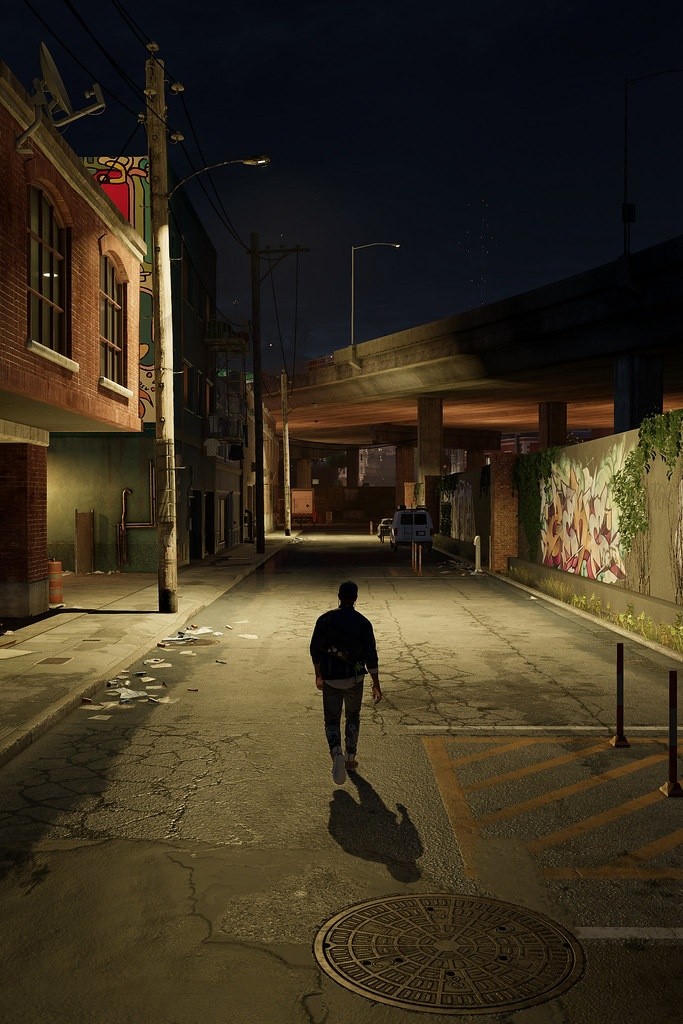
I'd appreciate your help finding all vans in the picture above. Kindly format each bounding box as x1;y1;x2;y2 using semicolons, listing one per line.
389;504;435;551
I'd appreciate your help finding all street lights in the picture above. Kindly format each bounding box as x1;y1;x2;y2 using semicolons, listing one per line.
346;238;406;357
164;155;278;195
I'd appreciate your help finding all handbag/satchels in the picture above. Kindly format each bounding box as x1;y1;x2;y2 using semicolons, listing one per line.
321;635;354;663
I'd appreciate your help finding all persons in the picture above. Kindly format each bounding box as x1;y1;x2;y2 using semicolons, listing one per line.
309;580;383;785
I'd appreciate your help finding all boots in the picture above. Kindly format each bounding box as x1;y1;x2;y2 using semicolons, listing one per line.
345;750;358;770
333;746;346;785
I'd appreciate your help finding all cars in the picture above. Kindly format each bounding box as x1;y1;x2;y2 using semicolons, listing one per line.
377;518;395;537
